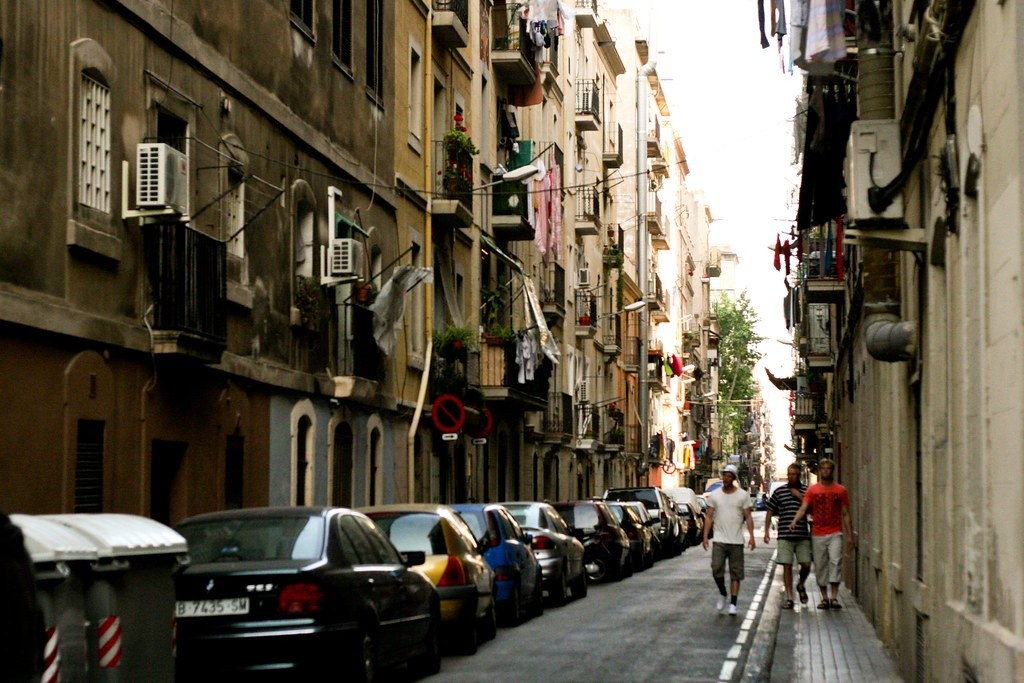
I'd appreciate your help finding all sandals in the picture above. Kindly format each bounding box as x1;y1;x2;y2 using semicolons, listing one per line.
830;598;841;609
817;599;829;609
797;584;808;603
783;599;794;609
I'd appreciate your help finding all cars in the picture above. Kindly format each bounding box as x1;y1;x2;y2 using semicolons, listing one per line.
169;504;445;682
497;499;589;607
446;501;543;629
549;485;770;584
353;505;498;657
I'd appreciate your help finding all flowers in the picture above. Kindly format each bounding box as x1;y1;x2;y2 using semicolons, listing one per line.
437;162;475;186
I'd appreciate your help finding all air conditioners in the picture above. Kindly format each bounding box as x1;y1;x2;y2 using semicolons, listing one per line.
806;301;830;356
843;118;903;221
794;393;814;417
578;380;590;402
332;237;364;277
135;143;190;213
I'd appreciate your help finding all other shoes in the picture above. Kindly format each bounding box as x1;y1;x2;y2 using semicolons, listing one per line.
728;604;737;615
717;594;728;610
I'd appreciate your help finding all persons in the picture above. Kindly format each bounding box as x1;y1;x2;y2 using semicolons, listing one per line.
789;458;854;610
764;464;810;609
703;464;756;615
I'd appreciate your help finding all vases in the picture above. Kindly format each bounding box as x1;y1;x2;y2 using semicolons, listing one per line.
445;178;467;192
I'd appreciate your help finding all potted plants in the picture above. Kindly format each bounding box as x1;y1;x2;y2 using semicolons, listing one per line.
484;325;513;346
444;130;479;161
433;322;474;352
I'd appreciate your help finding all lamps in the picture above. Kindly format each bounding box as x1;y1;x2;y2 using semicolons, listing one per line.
471;164;539;191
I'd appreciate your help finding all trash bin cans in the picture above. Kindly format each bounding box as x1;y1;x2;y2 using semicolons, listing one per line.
10;510;194;683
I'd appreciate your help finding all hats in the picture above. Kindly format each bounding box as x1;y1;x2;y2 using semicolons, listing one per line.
720;464;738;480
